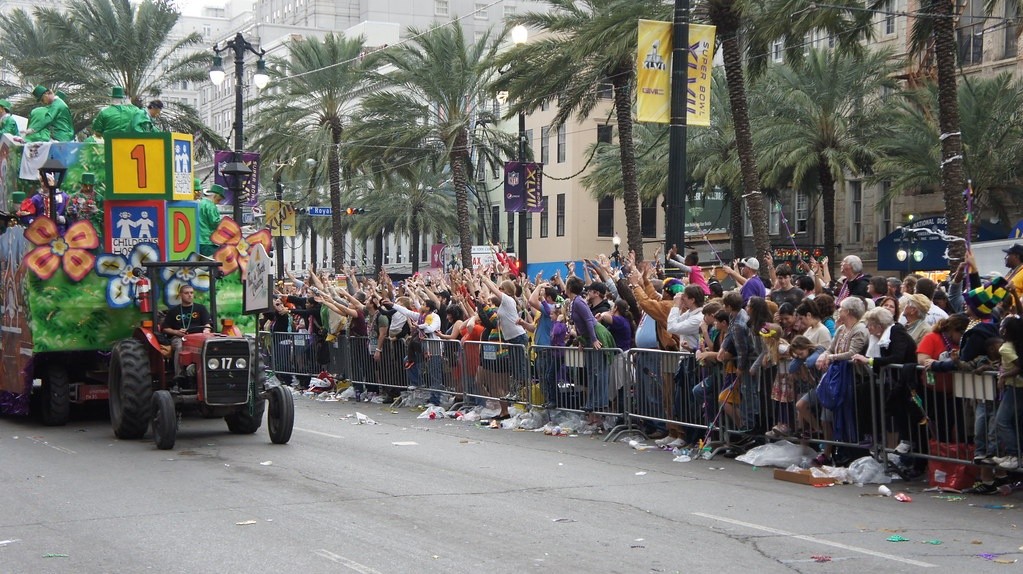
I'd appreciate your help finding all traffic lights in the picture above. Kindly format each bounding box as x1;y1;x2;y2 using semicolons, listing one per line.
346;207;364;215
294;207;306;215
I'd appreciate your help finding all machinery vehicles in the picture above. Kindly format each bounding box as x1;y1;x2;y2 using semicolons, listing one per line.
107;260;296;450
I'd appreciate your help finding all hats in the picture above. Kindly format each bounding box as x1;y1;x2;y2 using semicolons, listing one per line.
108;87;124;98
0;99;12;113
738;257;760;271
434;290;450;300
206;184;226;199
78;174;98;185
962;277;1009;318
663;277;685;296
33;84;49;102
309;285;319;296
904;292;932;315
1003;243;1023;255
584;282;606;294
194;179;203;191
12;192;26;202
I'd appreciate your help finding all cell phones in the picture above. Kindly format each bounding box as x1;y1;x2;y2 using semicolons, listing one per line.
434;330;438;333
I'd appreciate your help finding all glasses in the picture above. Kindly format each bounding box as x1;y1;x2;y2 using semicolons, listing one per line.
499;287;502;290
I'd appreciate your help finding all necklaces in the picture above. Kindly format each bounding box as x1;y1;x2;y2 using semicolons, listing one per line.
180;303;194;341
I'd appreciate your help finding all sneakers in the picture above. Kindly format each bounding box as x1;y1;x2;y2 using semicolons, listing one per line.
995;475;1009;492
667;437;688;448
962;479;997;495
655;435;676;446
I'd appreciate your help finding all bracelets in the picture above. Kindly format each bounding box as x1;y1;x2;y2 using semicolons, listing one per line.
869;358;874;366
375;348;382;352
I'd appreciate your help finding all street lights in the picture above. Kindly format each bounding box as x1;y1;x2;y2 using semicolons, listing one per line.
896;214;924;274
209;31;271;227
511;23;529;279
612;231;621;267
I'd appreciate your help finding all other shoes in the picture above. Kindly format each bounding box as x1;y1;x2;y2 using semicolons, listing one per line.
992;455;1018;469
894;440;911;454
812;453;832;467
492;414;510;420
170;381;180;394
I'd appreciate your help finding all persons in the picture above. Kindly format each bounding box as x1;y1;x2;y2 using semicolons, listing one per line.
84;87;163;142
0;84;74;142
8;191;26;216
1008;220;1023;239
194;177;203;201
16;173;68;237
162;285;216;394
68;172;104;239
259;241;1023;495
197;184;227;256
5;216;18;227
